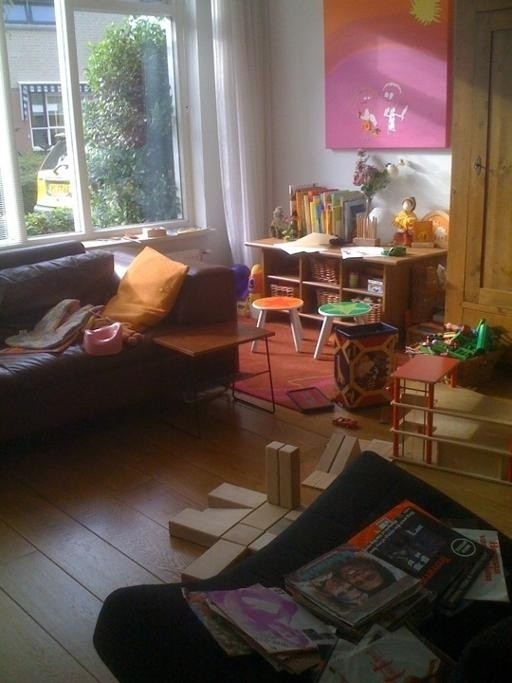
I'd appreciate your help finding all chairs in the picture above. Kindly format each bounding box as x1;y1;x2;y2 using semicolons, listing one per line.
92;451;512;683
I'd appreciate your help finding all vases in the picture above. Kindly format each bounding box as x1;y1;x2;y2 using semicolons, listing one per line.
352;211;381;247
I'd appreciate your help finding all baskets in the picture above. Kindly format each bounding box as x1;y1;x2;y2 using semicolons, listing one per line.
405;339;505;388
270;254;384;325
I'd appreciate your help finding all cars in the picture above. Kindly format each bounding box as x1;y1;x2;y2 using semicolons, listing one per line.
32;130;122;221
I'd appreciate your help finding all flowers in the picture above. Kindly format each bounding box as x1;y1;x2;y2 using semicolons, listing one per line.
352;147;388;211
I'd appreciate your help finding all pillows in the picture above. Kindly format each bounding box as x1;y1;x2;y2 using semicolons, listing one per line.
98;247;189;333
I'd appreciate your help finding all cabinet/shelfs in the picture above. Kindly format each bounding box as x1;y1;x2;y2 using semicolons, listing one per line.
245;236;447;341
391;354;512;488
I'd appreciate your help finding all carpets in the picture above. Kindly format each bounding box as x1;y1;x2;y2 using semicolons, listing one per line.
230;309;339;413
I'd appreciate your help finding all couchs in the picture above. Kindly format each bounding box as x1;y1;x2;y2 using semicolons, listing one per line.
1;242;250;446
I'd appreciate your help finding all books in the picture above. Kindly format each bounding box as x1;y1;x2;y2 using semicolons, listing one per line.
290;185;366;243
286;541;434;644
181;583;336;681
349;502;508;606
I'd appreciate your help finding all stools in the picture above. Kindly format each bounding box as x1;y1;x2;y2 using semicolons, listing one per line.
314;301;372;359
250;297;304;353
